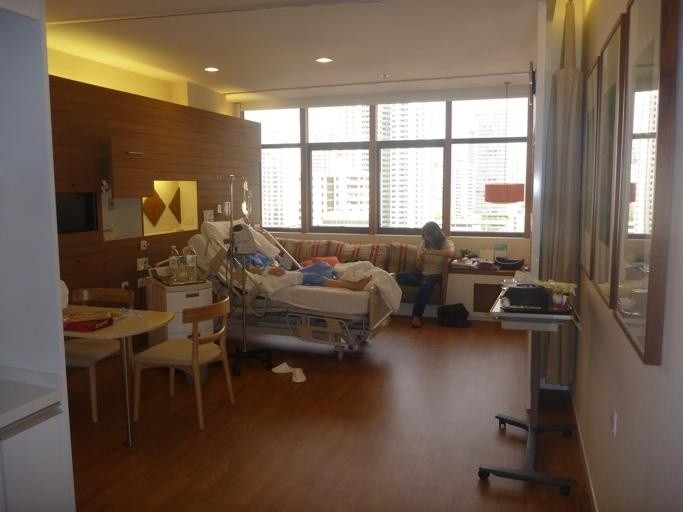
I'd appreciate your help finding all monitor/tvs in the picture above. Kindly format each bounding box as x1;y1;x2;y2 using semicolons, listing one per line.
55;192;98;234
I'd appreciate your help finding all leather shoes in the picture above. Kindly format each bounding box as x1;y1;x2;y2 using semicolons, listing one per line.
411;318;423;328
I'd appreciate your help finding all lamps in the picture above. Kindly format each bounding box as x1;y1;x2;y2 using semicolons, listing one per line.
482;180;524;268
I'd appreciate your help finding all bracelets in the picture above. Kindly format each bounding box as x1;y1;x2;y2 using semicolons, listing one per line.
428;249;432;255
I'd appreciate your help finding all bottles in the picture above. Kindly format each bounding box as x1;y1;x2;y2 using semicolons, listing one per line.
242;190;252;219
186;244;198;283
169;245;179;280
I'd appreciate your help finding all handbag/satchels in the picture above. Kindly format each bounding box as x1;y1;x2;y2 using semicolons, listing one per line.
437;303;472;326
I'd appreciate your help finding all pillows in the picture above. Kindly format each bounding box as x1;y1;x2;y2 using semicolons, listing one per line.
200;216;269;246
273;238;418;275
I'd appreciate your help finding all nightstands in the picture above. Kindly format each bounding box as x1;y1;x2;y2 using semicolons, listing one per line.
144;277;215;348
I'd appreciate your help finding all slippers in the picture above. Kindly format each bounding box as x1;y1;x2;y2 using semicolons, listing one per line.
292;367;306;384
271;362;293;374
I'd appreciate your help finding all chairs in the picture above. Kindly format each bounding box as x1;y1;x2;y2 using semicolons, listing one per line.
67;284;237;449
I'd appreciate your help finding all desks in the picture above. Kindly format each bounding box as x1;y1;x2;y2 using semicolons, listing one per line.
445;258;528;319
475;279;582;497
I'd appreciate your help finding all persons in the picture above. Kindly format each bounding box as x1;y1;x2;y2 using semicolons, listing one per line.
393;221;456;329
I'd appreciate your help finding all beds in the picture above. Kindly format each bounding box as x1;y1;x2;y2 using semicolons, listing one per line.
169;219;398;379
